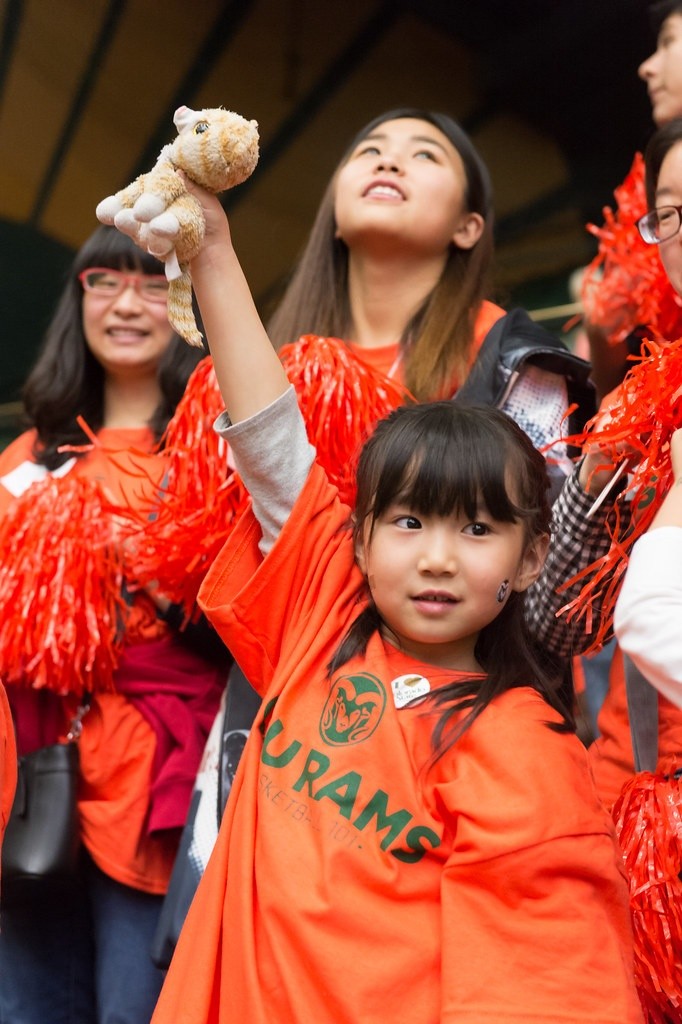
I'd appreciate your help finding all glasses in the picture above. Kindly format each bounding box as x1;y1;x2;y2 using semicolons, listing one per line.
635;205;682;245
80;268;169;304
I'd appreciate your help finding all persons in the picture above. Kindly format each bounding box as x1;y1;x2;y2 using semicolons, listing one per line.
0;9;680;1022
153;181;649;1024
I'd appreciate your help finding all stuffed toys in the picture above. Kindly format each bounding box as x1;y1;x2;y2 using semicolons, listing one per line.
95;104;260;348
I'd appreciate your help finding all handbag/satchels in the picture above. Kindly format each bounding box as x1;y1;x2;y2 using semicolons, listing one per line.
2;745;83;879
